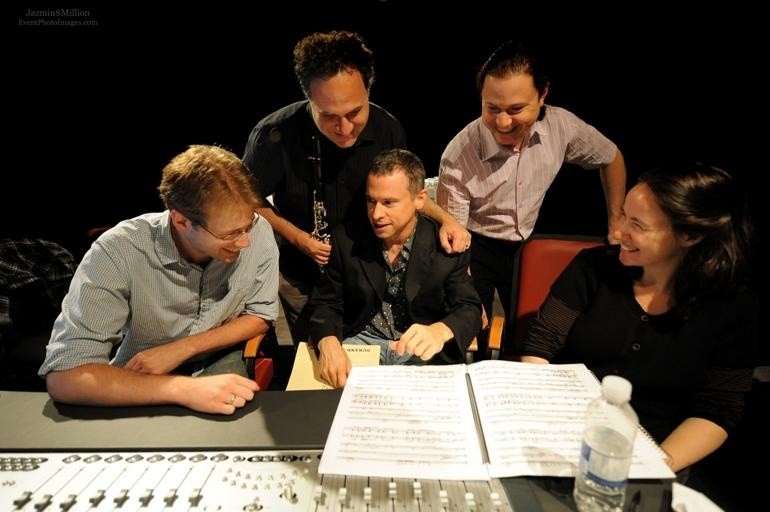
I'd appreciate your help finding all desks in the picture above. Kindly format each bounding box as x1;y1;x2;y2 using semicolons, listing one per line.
0;388;677;512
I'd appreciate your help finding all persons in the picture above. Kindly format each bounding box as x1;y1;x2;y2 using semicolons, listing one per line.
302;148;483;389
435;46;628;362
241;31;409;343
38;144;284;416
520;162;759;493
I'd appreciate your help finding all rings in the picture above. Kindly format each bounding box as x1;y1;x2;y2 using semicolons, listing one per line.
226;393;236;403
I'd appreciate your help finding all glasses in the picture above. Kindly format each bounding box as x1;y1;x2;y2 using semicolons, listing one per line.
194;211;260;240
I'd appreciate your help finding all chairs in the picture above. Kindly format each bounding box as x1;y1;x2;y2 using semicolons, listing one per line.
466;338;479;365
502;234;608;360
242;326;284;391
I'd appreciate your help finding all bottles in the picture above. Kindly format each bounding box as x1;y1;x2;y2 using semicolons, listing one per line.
571;371;641;512
